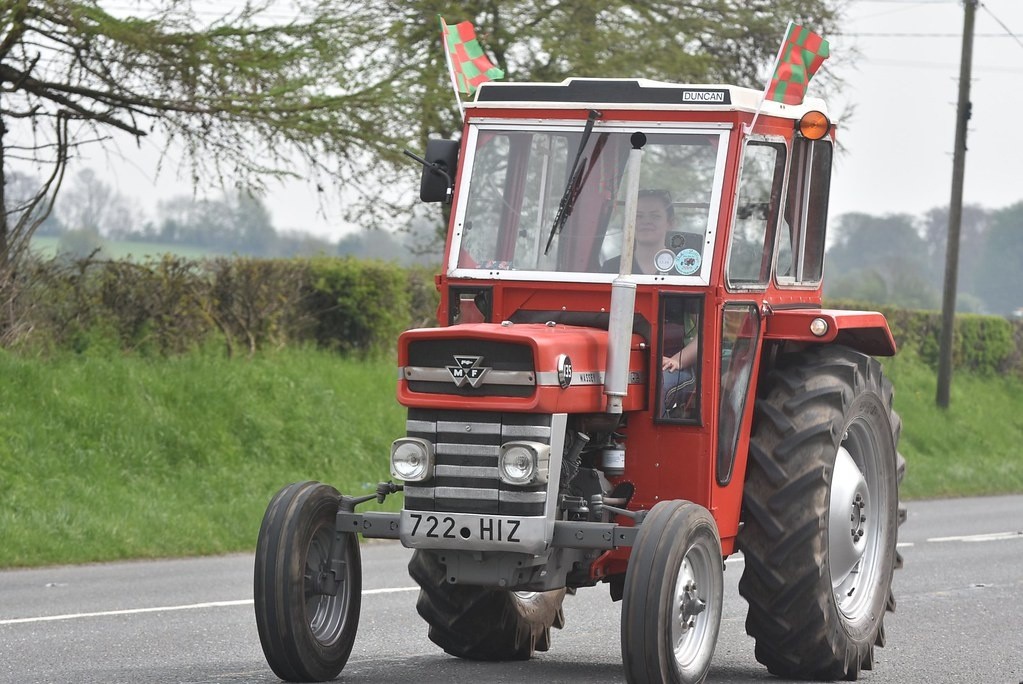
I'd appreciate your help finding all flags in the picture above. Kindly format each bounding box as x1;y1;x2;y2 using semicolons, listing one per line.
438;17;507;96
764;23;830;106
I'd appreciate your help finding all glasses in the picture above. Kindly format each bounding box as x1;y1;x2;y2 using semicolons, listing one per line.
638;189;673;204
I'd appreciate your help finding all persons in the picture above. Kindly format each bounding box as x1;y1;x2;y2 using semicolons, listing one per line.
600;189;698;416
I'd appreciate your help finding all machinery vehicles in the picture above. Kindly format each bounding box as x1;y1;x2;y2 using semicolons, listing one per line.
254;77;909;684
